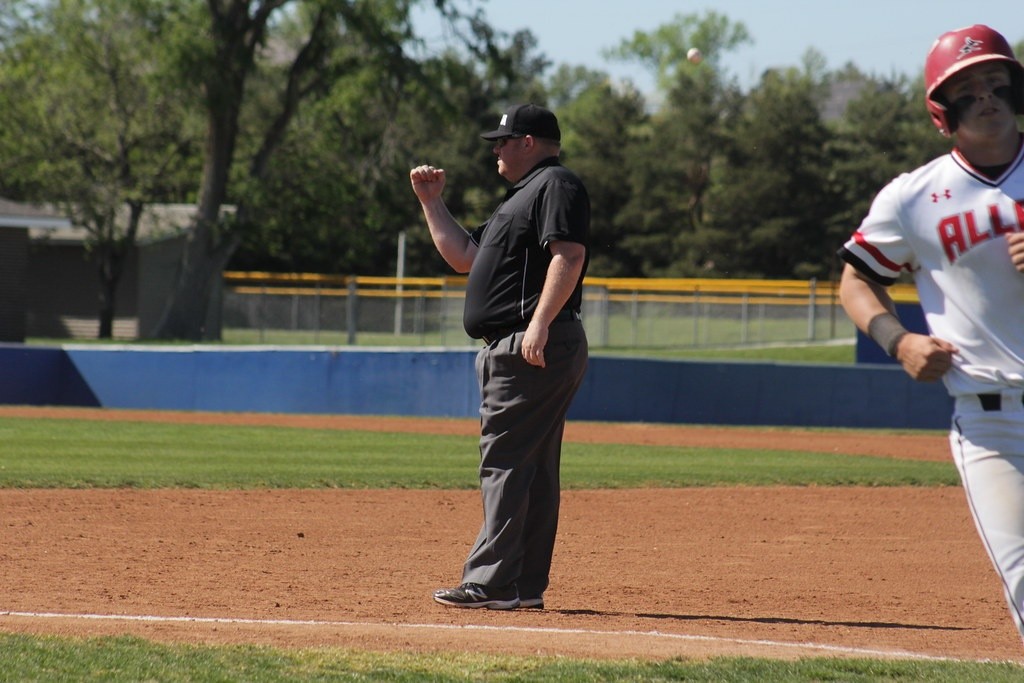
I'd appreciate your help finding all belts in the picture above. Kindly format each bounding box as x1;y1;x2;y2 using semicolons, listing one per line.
976;394;1003;412
481;312;581;345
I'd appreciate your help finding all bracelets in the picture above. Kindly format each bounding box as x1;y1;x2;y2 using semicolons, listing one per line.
869;313;907;359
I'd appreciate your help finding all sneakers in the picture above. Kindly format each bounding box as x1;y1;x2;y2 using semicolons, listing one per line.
519;593;544;607
433;582;520;610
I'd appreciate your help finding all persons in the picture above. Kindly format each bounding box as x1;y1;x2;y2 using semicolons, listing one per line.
408;103;587;613
837;24;1024;648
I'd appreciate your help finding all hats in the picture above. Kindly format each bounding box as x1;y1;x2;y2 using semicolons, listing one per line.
480;102;561;141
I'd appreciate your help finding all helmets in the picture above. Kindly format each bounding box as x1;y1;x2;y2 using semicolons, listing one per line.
926;25;1024;139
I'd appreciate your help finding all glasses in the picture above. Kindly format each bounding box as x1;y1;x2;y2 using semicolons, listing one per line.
497;136;524;147
